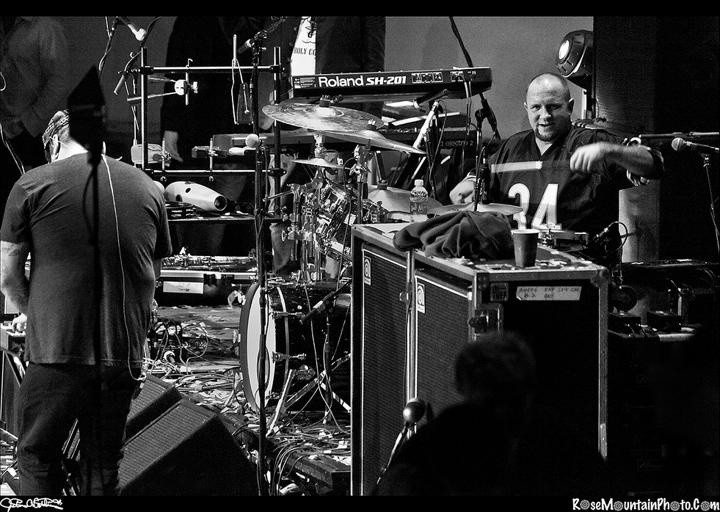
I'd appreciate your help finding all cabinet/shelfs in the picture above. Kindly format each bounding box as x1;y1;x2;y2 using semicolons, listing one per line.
347;220;613;497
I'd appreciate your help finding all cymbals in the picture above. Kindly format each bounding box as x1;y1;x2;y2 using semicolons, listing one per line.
292;159;351;171
262;102;384;133
349;185;447;222
427;201;522;217
306;129;427;154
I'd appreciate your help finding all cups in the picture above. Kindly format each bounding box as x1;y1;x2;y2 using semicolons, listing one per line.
510;228;541;268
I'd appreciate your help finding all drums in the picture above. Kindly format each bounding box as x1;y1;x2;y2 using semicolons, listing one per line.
238;278;351;417
303;181;392;269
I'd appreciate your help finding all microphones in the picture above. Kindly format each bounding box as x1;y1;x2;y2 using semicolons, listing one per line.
238;34;258;55
481;149;491;205
671;138;719;154
175;79;188;96
117;15;146;41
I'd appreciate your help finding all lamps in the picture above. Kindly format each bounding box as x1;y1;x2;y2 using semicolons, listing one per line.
555;29;594;119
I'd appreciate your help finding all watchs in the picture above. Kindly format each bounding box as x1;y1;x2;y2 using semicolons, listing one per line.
457;191;471;203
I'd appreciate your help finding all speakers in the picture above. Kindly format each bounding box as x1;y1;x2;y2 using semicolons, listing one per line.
114;399;254;499
63;376;182;473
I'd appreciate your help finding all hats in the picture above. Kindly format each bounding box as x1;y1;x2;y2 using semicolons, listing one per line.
41;108;69;162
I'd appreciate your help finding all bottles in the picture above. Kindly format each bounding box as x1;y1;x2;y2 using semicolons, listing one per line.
409;178;428;223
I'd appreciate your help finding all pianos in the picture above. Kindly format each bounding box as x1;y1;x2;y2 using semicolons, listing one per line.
270;68;493;105
1;319;26;353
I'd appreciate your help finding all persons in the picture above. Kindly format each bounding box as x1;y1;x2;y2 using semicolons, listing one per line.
159;16;266;308
0;109;173;497
369;330;608;494
449;72;665;365
0;17;73;197
267;16;386;281
